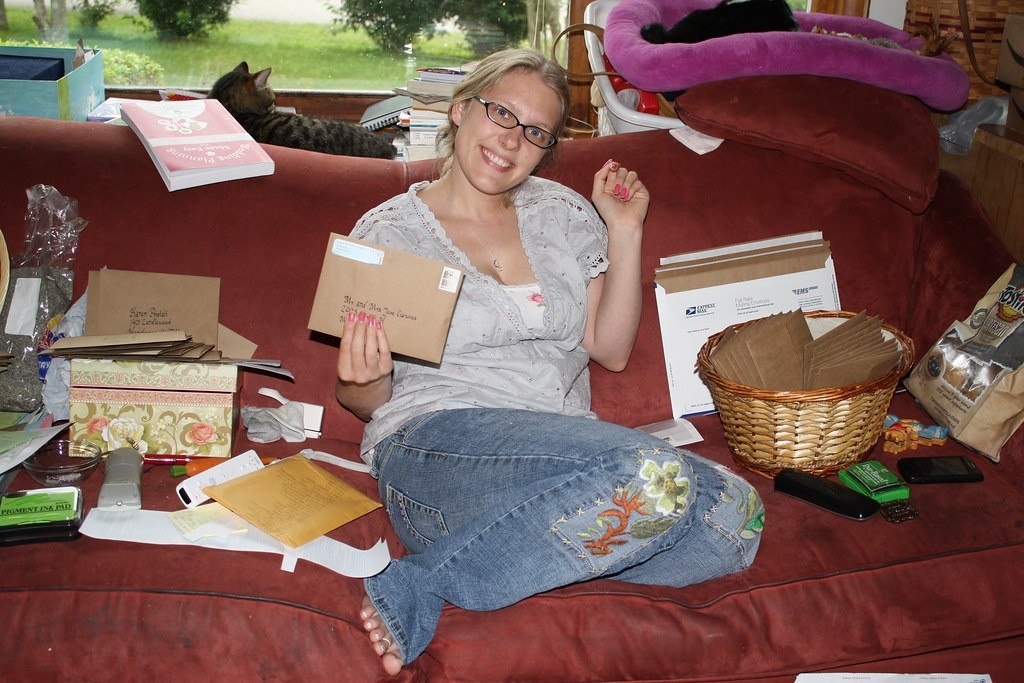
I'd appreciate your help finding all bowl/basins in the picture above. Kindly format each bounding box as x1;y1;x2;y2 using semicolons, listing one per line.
21;439;103;487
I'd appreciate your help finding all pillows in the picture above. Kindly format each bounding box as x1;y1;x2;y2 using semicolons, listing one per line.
675;75;942;214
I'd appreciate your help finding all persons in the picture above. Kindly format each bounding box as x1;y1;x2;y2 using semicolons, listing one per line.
335;48;765;676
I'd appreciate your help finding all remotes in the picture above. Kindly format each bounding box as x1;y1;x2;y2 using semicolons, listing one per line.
97;447;145;510
773;468;881;520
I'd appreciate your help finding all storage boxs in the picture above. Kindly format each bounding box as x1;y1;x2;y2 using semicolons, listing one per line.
583;0;685;133
69;357;243;459
0;45;105;121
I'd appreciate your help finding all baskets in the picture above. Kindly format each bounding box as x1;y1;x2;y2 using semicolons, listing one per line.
694;309;914;477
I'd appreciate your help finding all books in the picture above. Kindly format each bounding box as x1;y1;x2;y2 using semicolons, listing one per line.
359;67;475;162
121;99;275;192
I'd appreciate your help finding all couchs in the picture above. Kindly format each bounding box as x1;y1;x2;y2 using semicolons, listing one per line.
0;116;1024;682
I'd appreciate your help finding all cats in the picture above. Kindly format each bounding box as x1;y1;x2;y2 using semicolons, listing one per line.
205;61;397;160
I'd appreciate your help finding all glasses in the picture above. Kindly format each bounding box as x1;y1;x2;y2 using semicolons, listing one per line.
475;94;561;149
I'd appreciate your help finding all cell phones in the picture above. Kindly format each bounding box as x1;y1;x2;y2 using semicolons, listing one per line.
897;455;984;484
